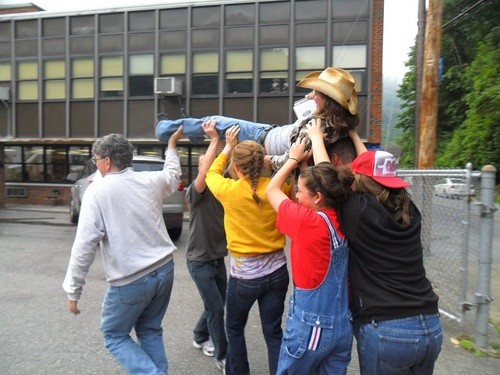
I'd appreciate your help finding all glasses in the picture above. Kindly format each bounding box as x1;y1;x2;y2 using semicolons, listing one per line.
91;155;104;164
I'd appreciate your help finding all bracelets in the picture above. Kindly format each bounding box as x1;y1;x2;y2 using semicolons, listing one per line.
289;158;300;164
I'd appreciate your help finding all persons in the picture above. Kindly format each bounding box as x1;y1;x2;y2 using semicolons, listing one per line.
156;67;360;172
63;124;186;375
184;118;443;375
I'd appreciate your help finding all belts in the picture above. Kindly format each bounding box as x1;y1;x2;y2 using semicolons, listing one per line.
259;123;278;144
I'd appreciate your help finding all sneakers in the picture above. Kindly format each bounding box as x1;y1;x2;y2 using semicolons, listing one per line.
214;358;227;375
192;338;215;357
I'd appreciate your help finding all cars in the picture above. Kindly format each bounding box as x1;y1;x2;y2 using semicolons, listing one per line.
435;178;476;202
66;155;185;239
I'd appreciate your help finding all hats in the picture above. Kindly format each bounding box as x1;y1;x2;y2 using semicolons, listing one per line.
295;66;359;116
351;149;412;190
